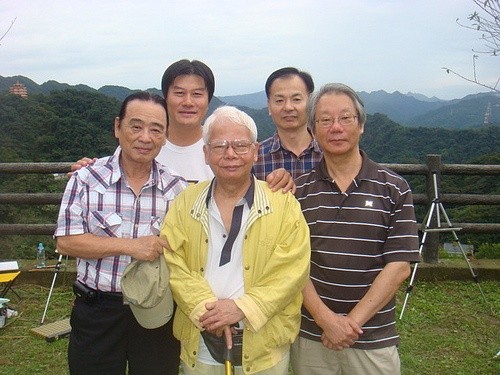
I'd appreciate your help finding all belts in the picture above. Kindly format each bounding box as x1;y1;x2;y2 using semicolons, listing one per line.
95;292;123;305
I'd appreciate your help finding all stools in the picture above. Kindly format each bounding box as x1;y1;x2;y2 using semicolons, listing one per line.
0;271;21;300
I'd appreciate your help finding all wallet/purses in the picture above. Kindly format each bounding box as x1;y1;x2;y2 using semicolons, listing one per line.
198;324;244;365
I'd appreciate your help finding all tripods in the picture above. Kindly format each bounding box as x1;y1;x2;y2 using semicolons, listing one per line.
397;170;488;319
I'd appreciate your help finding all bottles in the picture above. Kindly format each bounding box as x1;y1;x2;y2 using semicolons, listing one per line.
36;243;45;269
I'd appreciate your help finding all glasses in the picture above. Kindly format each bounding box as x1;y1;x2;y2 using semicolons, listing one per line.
209;138;253;155
314;111;359;129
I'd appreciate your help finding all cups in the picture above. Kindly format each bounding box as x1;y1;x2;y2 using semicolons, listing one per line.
0;299;10;328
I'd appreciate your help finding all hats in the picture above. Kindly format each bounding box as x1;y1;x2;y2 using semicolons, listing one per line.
118;252;174;329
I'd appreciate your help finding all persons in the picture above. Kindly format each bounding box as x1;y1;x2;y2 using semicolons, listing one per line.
251;67;324;182
53;92;296;375
290;83;422;375
70;59;216;188
159;105;311;375
